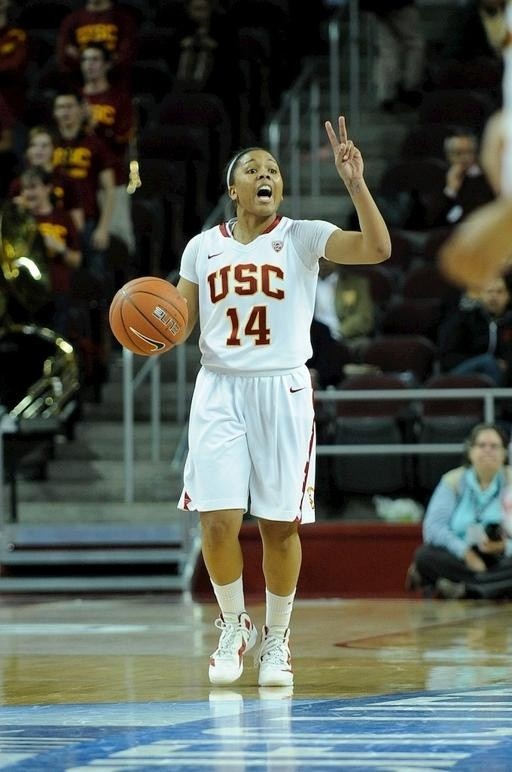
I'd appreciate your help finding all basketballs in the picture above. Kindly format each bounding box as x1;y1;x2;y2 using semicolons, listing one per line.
109;276;188;356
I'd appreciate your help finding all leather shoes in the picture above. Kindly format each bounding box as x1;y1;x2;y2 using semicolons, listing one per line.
404;563;424;592
435;578;487;601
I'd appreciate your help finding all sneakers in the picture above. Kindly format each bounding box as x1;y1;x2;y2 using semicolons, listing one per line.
258;624;294;687
208;611;258;685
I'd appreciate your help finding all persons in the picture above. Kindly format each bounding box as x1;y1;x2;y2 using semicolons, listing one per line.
407;420;510;598
1;0;509;515
176;115;392;690
